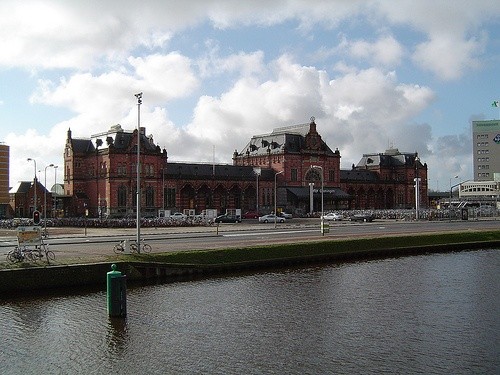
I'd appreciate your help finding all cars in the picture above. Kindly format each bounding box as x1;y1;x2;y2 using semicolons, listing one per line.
281;212;290;219
144;214;155;219
321;213;344;221
215;215;242;224
170;212;186;219
258;214;286;223
350;213;373;222
242;211;260;219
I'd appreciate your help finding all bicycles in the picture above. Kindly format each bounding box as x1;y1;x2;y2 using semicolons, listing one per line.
113;237;152;254
5;236;57;264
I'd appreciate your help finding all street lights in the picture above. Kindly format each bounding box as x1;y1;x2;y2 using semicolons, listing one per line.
313;165;324;233
274;170;285;229
449;176;460;222
27;158;38;211
43;164;54;237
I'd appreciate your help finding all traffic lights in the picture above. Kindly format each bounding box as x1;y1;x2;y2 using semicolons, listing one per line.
33;211;40;225
